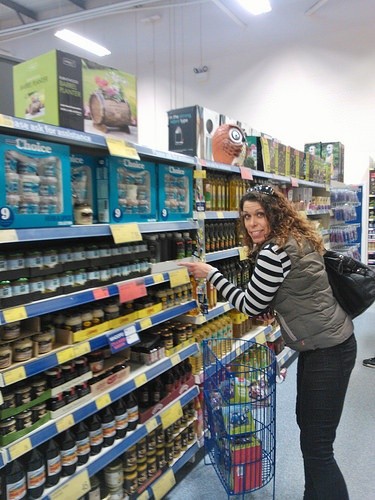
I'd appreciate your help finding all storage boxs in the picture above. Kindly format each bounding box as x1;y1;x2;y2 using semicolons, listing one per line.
221;377;248;406
214;432;262;494
218;404;255;435
0;49;344;228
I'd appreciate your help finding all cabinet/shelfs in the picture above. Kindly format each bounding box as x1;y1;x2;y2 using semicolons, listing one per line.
0;114;375;500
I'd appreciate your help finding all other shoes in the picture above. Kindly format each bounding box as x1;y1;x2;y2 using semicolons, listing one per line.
362;357;375;367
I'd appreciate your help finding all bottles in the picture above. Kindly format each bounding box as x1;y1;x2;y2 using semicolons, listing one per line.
140;171;286;439
0;357;192;500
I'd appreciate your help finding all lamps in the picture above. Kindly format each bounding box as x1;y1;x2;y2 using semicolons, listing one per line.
193;66;208;83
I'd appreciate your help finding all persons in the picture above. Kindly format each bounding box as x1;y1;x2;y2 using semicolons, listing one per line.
177;186;358;500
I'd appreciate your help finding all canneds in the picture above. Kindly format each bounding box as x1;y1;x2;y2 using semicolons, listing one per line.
0;284;193;436
79;398;195;500
4;151;187;225
0;241;154;308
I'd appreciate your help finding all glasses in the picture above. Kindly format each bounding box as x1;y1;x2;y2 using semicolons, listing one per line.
246;186;279;198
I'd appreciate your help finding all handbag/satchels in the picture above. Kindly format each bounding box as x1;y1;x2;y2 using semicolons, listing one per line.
323;250;375;320
